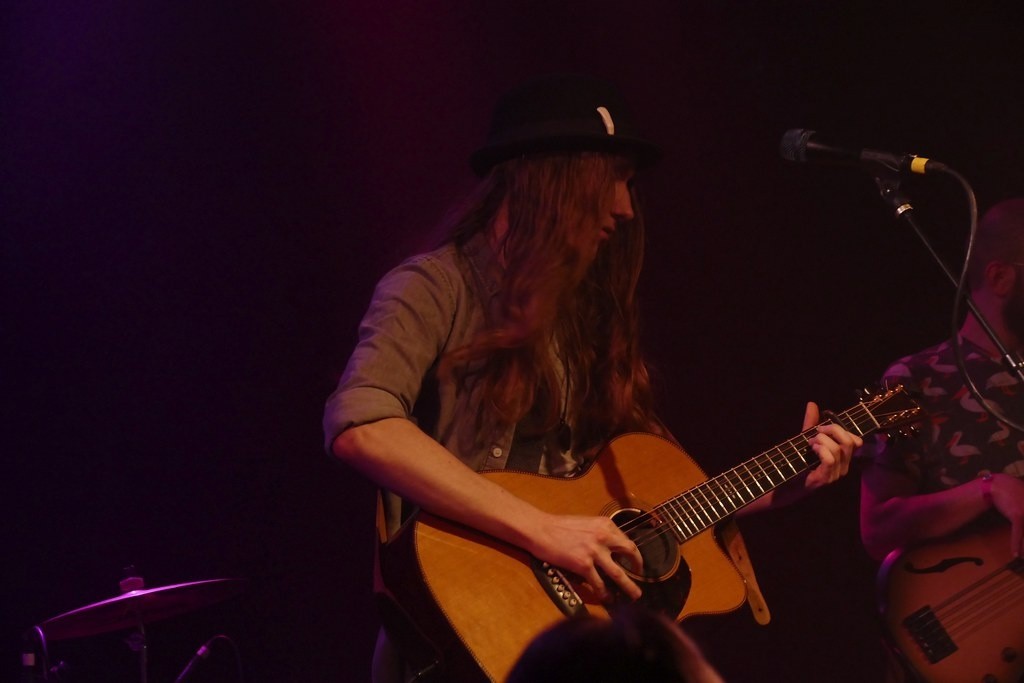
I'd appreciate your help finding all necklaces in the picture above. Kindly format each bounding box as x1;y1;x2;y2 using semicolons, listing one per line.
556;316;573;451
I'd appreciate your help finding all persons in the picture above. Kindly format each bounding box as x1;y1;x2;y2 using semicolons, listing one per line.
319;72;864;683
858;198;1024;683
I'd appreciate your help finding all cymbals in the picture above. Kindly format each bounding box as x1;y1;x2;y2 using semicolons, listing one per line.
23;574;250;642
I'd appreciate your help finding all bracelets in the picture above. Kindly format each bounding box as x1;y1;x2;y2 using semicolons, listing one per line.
983;471;992;491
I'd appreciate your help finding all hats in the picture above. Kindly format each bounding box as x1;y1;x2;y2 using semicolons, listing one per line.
471;65;662;170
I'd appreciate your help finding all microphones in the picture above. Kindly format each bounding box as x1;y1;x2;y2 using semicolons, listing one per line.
176;638;216;683
20;630;40;683
780;128;948;176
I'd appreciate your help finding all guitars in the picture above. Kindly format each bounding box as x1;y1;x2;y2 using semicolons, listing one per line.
375;377;928;683
868;473;1024;683
